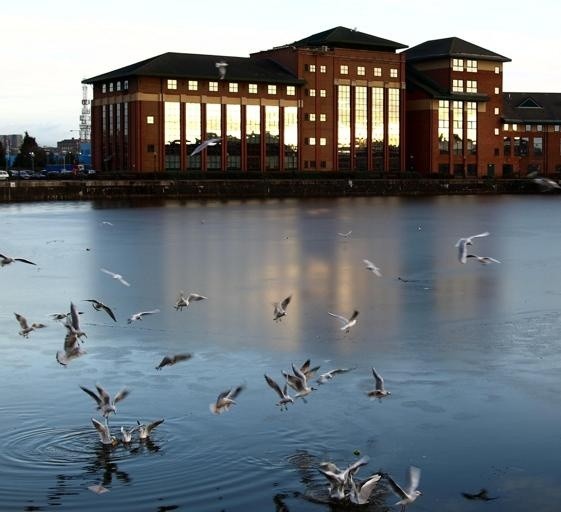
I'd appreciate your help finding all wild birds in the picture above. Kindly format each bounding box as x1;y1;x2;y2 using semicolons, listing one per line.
101;267;129;286
155;353;191;371
455;231;490;264
127;310;160;329
215;60;228;81
0;252;37;268
467;253;502;265
82;299;117;323
533;177;560;189
318;455;424;512
78;382;164;447
103;221;113;226
272;295;292;323
13;312;47;339
174;293;208;311
264;359;349;413
49;300;88;366
338;231;352;239
210;385;244;415
192;136;222;157
459;489;500;502
365;367;392;401
362;259;382;278
327;310;360;335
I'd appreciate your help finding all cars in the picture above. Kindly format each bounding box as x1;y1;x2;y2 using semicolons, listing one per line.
0;165;98;181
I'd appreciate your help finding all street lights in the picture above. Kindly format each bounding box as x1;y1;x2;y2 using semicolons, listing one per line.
70;128;82;165
29;151;34;176
62;150;66;175
